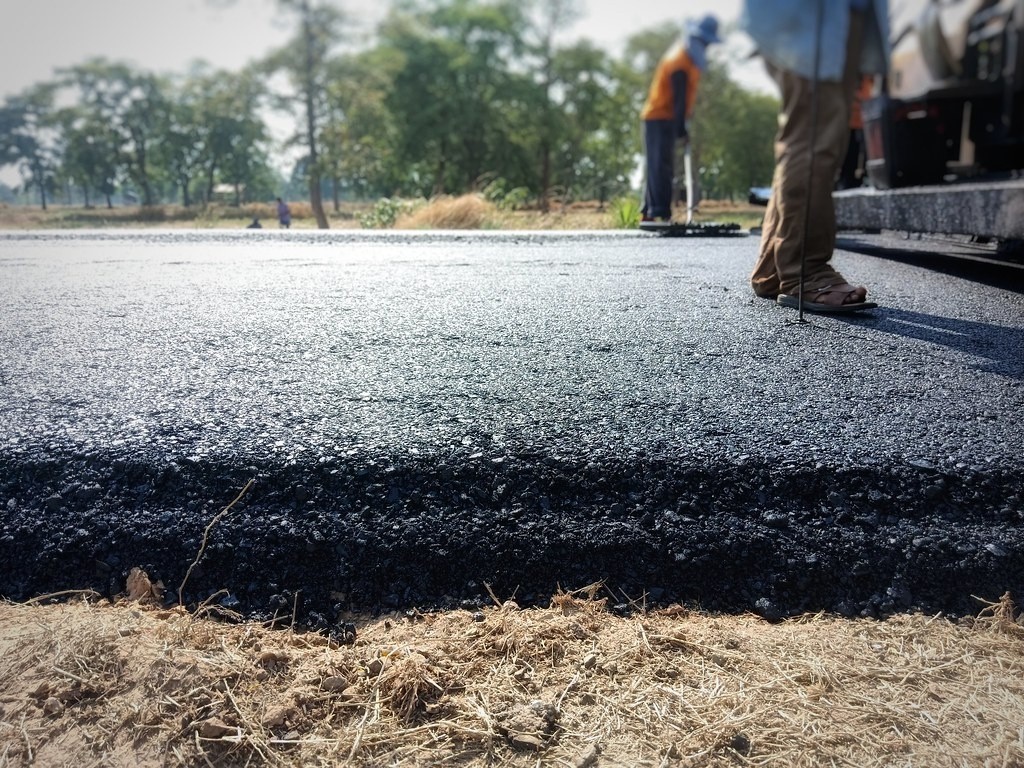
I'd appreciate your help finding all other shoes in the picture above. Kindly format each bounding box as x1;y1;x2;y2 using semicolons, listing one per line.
639;216;657;225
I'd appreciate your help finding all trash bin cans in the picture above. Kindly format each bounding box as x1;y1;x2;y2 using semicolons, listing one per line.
881;101;948;188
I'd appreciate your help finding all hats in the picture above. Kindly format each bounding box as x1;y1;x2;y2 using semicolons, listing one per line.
682;15;723;45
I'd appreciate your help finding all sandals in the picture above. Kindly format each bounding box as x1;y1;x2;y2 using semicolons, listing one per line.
776;281;878;314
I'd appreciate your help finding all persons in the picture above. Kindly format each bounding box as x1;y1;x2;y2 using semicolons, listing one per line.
638;16;724;224
276;197;291;228
743;0;892;313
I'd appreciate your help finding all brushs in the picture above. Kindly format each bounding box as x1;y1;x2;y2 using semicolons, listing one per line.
640;121;741;235
